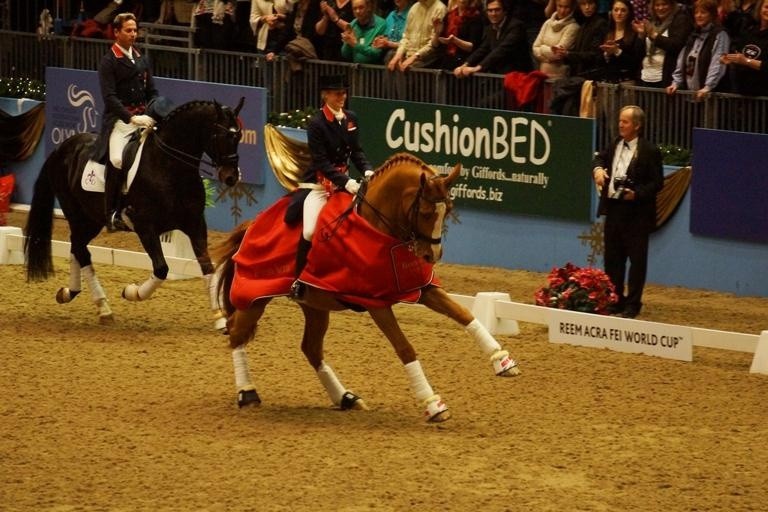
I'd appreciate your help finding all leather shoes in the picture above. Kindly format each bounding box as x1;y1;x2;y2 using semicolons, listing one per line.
623;304;643;318
606;299;627;314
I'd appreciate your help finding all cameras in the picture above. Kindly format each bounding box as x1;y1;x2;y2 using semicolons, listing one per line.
614;176;633;191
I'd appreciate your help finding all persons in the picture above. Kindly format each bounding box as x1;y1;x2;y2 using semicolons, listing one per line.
73;0;768;145
288;73;376;300
98;12;160;232
590;105;666;318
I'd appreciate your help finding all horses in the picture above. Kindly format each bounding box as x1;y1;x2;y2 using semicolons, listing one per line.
197;152;523;424
22;96;246;336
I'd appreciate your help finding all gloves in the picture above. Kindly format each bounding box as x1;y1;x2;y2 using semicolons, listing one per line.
344;178;361;195
130;114;158;127
364;169;375;182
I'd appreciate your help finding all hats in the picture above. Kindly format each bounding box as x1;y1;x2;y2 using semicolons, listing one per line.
318;74;352;90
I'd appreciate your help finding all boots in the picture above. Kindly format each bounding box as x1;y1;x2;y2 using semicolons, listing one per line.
103;159;125;233
290;232;312;299
